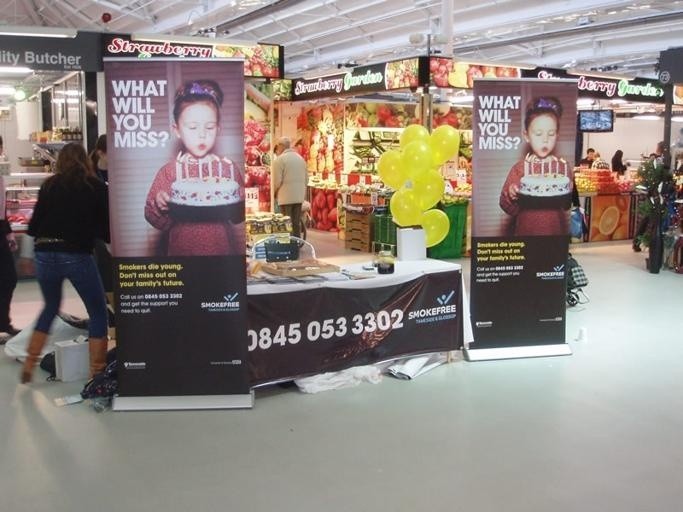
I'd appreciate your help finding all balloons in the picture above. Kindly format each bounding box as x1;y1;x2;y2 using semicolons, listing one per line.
378;124;460;249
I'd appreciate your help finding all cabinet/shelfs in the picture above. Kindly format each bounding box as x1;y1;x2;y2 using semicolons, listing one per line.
2;188;40;233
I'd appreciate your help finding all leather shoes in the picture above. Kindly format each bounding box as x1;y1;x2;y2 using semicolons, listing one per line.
0;324;21;335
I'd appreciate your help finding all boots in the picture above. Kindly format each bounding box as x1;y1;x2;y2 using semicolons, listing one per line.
21;330;48;385
87;336;107;382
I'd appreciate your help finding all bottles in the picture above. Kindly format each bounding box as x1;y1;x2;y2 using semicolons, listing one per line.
31;128;83;142
377;251;394;274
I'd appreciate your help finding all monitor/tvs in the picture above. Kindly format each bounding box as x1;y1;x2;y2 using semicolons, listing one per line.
577;109;614;133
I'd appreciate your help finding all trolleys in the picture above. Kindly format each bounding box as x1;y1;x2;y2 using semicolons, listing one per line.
566;253;590;306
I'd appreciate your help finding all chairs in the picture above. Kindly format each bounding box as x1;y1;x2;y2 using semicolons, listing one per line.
252;236;315;264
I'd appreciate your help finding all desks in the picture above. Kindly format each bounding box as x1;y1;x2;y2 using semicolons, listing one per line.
245;258;462;396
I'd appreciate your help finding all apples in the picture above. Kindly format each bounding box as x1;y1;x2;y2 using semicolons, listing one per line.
312;190;340;232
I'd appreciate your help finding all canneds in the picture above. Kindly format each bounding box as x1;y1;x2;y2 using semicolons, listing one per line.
246;213;293;234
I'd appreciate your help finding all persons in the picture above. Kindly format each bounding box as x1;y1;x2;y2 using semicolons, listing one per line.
21;143;109;382
144;80;246;257
87;135;115;339
499;97;575;239
578;142;665;252
272;137;309;244
0;135;23;345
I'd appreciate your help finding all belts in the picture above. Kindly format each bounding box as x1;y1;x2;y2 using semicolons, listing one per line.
33;237;64;245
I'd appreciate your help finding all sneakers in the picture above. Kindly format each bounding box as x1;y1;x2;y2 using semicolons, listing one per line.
632;244;641;251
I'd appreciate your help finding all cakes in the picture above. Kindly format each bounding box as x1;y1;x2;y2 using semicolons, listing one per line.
519;171;573;198
168;176;240;207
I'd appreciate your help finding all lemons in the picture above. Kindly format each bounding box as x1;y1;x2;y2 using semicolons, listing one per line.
575;178;598;193
